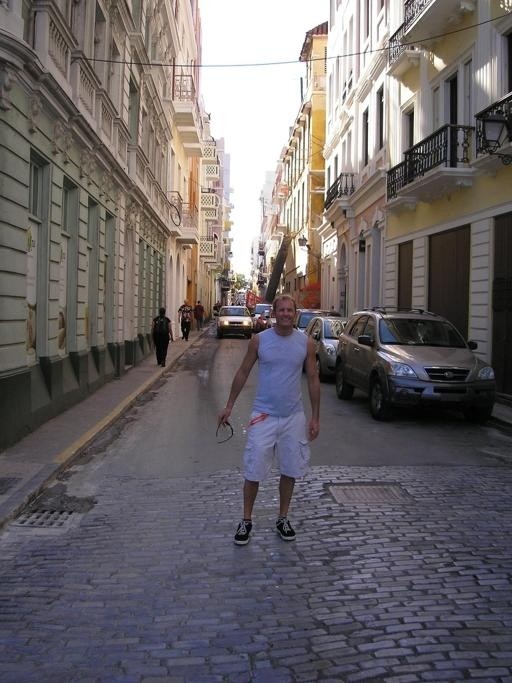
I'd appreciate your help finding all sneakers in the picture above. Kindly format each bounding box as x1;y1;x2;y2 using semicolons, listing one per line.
274;514;297;542
233;519;254;547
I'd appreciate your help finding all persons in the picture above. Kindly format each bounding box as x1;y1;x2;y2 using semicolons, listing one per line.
217;301;221;308
213;303;219;319
178;299;193;341
194;301;205;331
256;311;268;331
151;308;173;367
218;295;320;545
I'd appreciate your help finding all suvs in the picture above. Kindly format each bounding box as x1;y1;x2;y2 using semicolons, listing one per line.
333;305;502;427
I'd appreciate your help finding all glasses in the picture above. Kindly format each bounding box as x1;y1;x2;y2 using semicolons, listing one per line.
215;420;234;445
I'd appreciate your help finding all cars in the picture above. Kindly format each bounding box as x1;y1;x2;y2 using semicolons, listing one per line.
209;301;343;340
303;313;350;384
